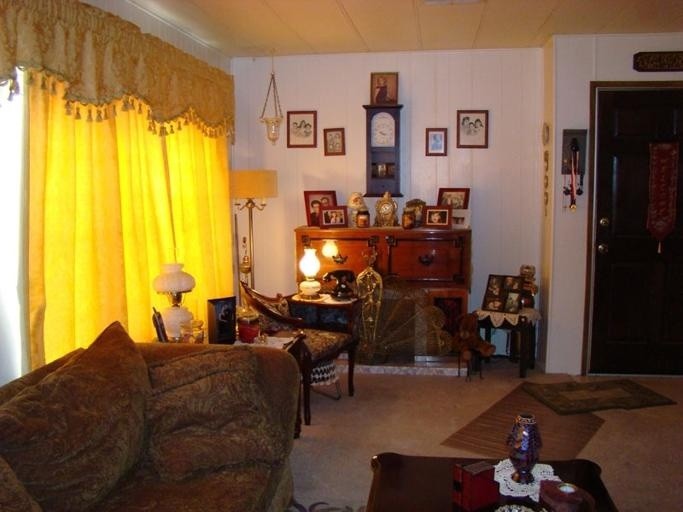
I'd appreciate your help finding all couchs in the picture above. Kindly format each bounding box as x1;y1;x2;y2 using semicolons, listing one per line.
1;321;301;512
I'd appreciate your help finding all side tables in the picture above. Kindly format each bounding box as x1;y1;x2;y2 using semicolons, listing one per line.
291;293;359;335
236;336;311;438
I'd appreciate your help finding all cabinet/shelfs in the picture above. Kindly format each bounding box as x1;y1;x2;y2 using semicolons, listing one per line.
293;223;472;293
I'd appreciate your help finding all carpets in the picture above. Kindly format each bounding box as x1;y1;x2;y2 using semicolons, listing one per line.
523;379;677;415
440;381;604;461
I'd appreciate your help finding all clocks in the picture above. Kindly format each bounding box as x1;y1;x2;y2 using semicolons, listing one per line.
362;104;403;197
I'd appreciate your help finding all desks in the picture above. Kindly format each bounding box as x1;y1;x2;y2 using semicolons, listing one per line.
473;307;536;378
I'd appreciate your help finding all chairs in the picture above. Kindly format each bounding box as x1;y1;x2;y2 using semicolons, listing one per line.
240;282;358;397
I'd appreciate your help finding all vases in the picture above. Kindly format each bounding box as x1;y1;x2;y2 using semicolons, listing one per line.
505;413;542;485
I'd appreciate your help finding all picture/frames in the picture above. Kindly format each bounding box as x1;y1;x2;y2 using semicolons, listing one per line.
324;128;345;156
437;187;469;209
287;111;316;148
422;206;452;230
370;72;398;104
319;206;348;228
426;128;447;156
304;191;337;227
457;110;488;149
482;274;524;314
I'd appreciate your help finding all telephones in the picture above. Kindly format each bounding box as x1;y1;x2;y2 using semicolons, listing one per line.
152;308;168;342
324;270;355;300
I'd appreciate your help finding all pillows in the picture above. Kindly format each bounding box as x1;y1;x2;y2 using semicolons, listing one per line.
146;344;262;389
146;370;287;483
1;321;152;512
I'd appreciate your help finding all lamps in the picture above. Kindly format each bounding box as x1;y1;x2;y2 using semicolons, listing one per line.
300;248;322;300
156;265;196;343
230;170;277;290
257;52;284;145
